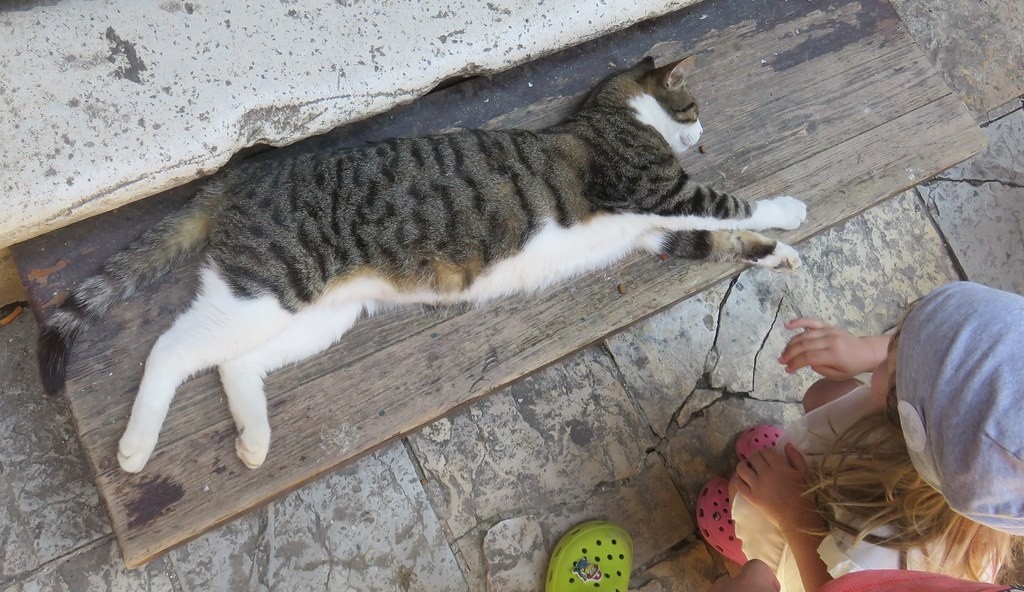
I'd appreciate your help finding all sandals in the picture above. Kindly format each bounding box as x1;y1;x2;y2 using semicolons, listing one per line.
735;424;787;464
545;519;633;592
696;476;750;566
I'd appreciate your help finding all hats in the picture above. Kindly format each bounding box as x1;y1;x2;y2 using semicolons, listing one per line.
895;280;1024;537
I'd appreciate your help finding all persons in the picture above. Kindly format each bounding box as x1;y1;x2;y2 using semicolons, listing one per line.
545;520;1024;592
696;281;1024;592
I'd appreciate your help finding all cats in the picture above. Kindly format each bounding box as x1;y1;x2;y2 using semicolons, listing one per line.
37;55;807;472
